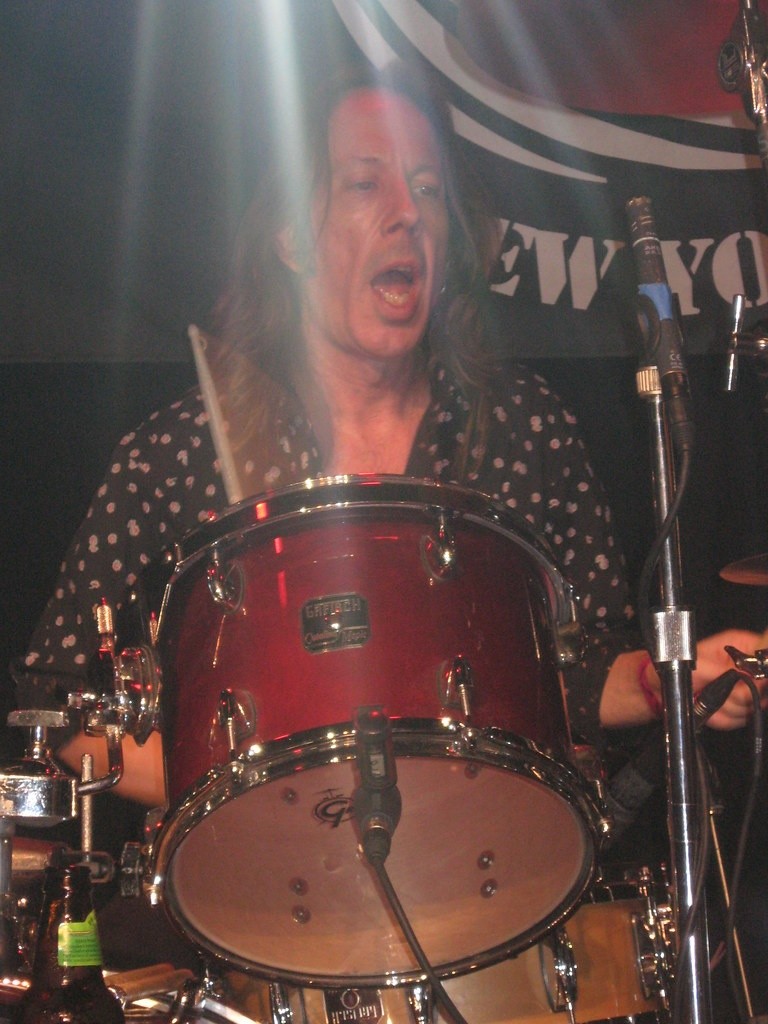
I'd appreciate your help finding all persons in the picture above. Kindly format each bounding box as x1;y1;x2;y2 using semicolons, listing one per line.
14;69;768;984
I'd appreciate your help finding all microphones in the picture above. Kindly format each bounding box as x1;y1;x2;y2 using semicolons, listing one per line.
603;667;740;849
625;196;695;453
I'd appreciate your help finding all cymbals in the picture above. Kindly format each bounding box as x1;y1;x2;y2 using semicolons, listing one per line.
719;552;768;585
455;0;768;118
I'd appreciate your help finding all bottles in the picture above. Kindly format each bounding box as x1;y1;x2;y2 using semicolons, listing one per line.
24;866;125;1024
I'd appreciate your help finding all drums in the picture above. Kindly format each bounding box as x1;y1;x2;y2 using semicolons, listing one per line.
144;471;607;990
202;864;680;1024
0;834;54;1005
